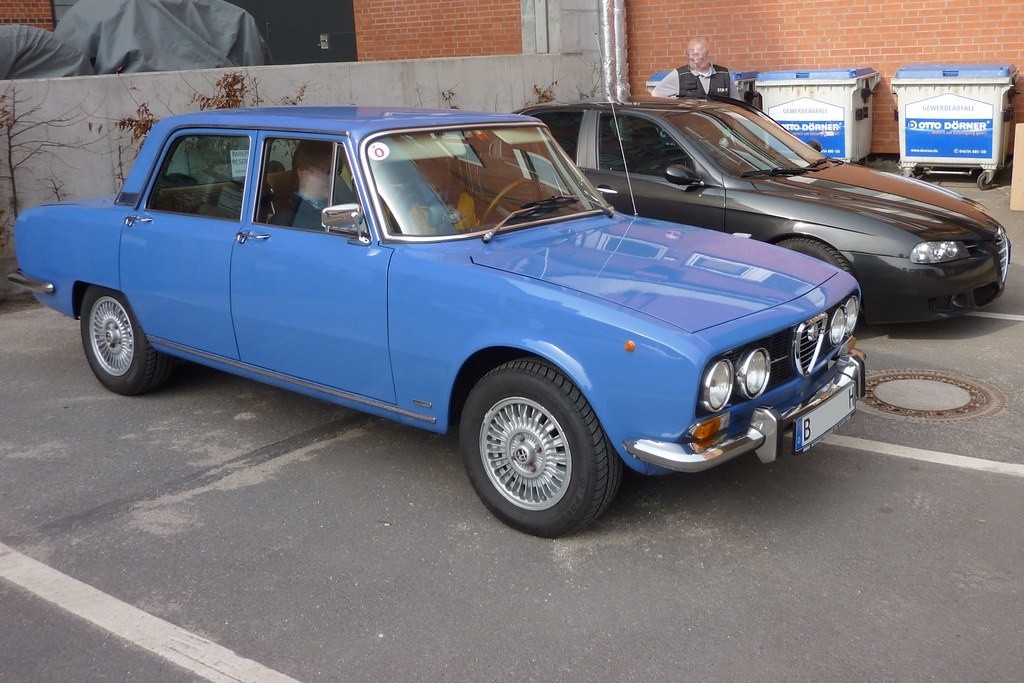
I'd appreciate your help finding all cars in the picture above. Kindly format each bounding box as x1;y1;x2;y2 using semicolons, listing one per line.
4;103;867;539
509;94;1012;327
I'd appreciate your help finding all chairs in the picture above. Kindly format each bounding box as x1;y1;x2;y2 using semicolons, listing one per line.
267;170;299;226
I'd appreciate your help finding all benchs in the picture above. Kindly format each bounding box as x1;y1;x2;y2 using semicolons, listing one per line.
153;181;244;220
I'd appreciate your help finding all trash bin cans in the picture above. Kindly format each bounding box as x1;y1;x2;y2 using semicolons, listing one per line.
892;63;1013;190
646;70;760;113
755;67;879;168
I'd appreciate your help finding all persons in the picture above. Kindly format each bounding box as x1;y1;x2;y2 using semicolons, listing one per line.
651;38;741;100
619;127;677;178
267;140;344;231
